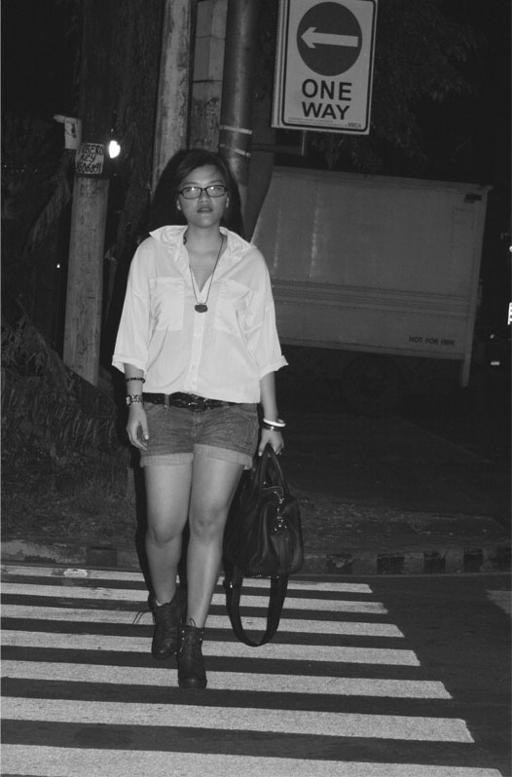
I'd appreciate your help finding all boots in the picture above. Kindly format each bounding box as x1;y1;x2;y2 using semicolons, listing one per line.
148;588;207;690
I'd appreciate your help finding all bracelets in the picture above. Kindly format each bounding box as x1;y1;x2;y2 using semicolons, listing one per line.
261;418;287;431
124;377;145;383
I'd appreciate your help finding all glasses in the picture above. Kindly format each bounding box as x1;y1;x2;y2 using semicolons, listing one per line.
176;185;230;199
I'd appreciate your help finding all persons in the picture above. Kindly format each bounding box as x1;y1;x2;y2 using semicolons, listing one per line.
110;148;286;696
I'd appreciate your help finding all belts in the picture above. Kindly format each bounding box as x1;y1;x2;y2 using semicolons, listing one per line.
142;392;241;413
225;470;304;578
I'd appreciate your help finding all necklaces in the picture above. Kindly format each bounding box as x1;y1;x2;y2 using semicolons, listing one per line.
184;232;224;312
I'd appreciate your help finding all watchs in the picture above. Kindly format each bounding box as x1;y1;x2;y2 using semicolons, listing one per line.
126;394;144;406
